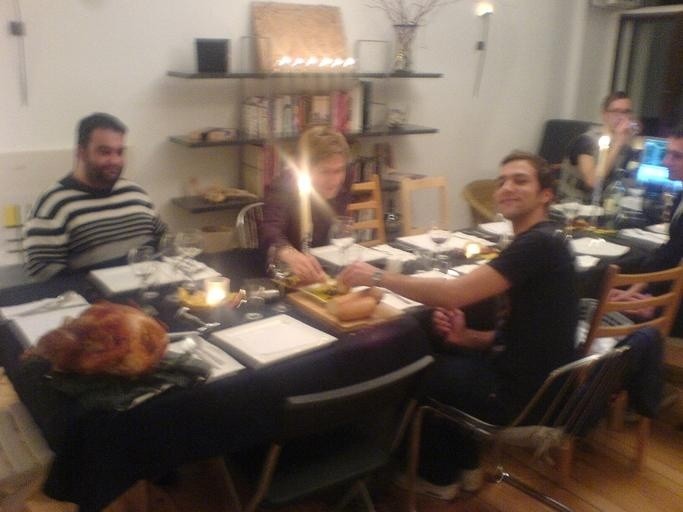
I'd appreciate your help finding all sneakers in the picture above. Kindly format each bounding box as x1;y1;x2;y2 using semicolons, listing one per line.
390;466;483;502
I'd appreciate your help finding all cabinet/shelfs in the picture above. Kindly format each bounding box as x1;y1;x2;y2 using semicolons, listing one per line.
168;70;443;212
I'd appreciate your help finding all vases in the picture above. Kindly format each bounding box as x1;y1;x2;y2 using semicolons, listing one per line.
393;24;418;70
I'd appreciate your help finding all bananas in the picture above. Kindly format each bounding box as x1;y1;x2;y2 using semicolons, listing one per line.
204;187;258;204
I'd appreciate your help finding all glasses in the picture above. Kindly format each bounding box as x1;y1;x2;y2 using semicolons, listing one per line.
608;109;634;117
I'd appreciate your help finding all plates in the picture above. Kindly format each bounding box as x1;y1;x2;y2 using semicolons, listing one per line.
9;196;675;411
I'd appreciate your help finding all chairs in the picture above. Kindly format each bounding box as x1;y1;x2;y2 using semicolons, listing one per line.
236;201;263;250
339;172;385;251
398;175;449;239
463;179;507;227
570;263;681;476
408;345;634;509
218;355;438;509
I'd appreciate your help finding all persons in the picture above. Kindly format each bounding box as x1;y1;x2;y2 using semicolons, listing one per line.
336;150;577;501
594;124;683;423
260;126;350;282
556;92;645;207
22;113;175;283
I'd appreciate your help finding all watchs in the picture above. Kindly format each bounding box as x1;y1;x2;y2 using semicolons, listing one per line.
371;268;384;286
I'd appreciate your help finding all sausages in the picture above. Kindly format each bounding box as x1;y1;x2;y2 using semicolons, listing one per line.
328;287;383;321
